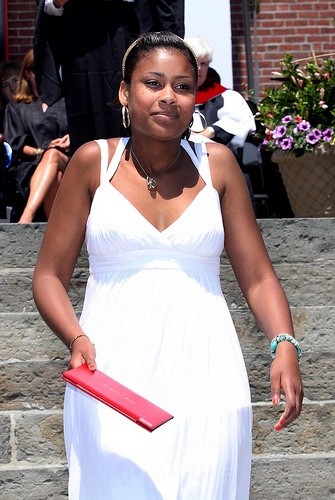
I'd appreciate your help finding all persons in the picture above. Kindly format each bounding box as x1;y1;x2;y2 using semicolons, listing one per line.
33;32;303;500
34;0;184;200
0;134;12;219
208;67;221;86
0;60;28;138
4;50;70;223
180;35;256;168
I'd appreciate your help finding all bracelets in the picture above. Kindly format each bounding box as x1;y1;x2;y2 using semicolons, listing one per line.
269;333;302;359
68;334;90;354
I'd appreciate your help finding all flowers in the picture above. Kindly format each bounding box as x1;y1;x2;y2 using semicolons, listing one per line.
253;51;335;152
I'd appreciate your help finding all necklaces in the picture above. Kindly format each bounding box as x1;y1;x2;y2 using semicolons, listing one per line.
131;143;181;189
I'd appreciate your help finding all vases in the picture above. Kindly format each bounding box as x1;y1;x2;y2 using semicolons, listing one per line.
260;142;335;219
